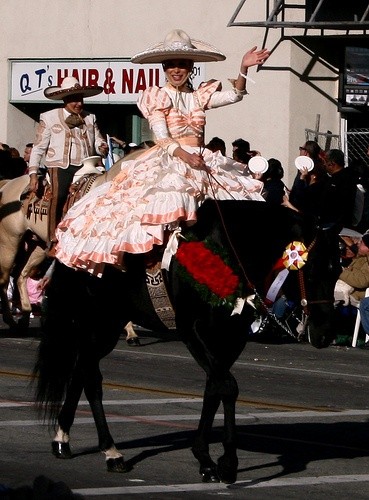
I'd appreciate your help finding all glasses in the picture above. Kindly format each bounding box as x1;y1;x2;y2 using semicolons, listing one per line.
299;146;305;152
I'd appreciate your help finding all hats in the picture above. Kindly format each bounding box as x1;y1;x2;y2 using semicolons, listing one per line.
131;29;226;63
44;76;104;100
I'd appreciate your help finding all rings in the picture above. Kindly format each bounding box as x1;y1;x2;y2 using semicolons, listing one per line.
193;162;196;166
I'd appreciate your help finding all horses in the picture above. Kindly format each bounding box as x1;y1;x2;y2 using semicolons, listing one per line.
0;142;151;349
23;199;346;484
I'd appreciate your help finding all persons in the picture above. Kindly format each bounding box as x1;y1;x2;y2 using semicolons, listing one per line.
109;136;137;155
23;142;34;174
204;136;369;349
28;77;109;234
54;29;270;278
